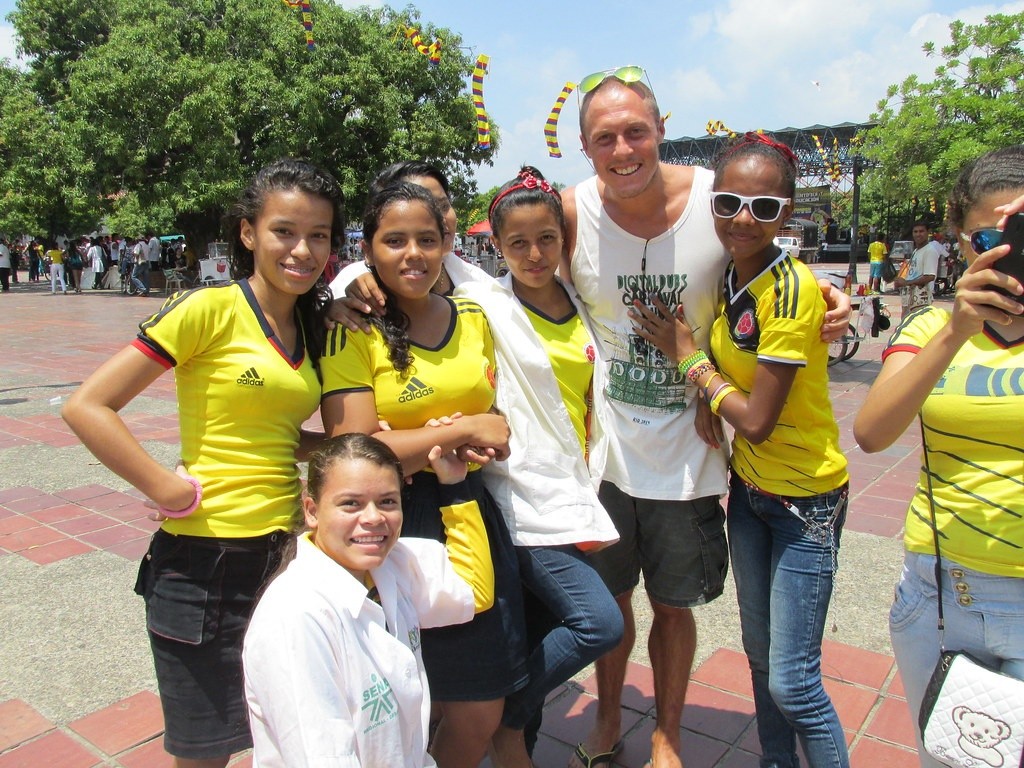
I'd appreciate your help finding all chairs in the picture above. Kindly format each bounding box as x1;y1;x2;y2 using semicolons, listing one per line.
161;267;184;296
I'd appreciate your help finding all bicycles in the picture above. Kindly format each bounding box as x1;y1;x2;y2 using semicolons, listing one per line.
44;266;59;283
817;277;890;367
120;262;138;296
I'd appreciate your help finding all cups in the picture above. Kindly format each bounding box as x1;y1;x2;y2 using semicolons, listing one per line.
49;396;62;405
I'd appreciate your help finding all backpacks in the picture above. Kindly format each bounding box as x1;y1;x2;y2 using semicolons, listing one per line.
168;242;181;263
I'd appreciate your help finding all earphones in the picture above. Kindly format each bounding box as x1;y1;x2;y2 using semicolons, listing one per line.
634;337;644;350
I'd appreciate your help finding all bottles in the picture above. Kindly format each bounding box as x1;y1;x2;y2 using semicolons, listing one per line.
845;273;851;289
859;285;864;295
845;287;851;296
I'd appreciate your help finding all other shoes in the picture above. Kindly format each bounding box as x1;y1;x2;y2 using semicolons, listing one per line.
91;286;104;290
64;291;67;295
138;293;150;297
869;290;882;295
76;290;81;295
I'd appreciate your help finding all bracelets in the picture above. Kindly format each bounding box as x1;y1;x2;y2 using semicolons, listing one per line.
676;348;737;414
158;472;207;519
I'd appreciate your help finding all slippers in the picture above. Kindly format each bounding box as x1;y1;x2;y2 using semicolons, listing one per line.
575;736;624;768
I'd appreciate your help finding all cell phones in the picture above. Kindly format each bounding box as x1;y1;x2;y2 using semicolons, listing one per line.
980;212;1024;317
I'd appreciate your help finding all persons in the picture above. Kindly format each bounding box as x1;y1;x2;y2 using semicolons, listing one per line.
0;159;625;768
629;130;854;767
849;148;1024;768
558;68;853;768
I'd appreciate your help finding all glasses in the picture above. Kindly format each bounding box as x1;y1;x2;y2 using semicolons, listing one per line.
576;66;655;114
709;189;791;223
957;228;1004;258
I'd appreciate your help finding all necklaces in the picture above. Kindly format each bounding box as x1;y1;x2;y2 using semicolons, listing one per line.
438;265;446;293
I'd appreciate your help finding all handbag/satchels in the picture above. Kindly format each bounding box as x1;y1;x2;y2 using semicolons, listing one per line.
917;651;1024;768
899;284;927;320
101;246;108;267
117;254;127;275
